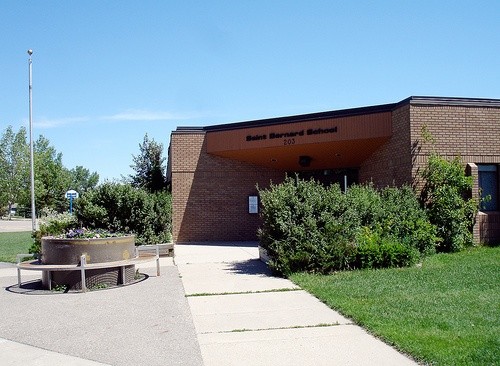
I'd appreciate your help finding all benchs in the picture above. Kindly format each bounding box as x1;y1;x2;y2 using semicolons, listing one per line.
16;250;161;292
